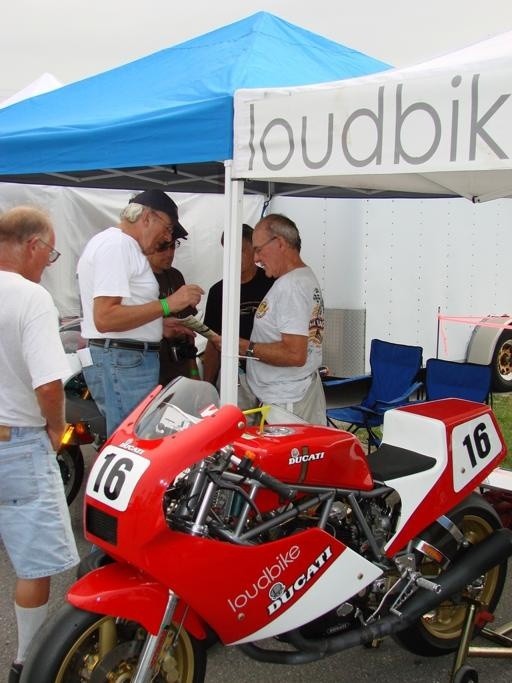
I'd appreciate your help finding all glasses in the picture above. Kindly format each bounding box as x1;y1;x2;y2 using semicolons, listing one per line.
148;209;178;237
26;234;61;263
253;235;279;254
154;238;180;253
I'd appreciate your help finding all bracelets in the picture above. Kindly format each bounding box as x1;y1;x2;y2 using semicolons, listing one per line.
161;299;172;318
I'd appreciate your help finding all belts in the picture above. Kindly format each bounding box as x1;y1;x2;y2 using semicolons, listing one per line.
87;336;166;352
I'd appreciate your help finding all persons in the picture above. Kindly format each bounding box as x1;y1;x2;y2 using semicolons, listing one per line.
202;224;275;424
144;214;200;390
75;188;205;444
211;214;327;431
0;204;68;683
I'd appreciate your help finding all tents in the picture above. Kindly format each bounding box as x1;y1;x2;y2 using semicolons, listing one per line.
0;1;399;404
0;72;65;111
228;2;512;410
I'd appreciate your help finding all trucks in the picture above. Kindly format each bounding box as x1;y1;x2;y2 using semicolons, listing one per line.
265;197;512;396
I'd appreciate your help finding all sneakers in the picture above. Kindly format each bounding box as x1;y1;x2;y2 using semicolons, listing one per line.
8;660;23;682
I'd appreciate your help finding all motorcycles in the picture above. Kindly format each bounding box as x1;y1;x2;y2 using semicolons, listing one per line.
19;375;512;683
53;317;108;507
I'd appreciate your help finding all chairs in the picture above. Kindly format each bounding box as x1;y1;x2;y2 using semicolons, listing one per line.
376;358;493;416
326;339;422;454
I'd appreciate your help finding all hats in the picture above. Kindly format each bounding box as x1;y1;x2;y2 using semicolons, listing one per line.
175;223;188;239
129;187;181;220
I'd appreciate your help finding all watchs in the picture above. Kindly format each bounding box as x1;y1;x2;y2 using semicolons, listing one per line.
246;339;255;357
